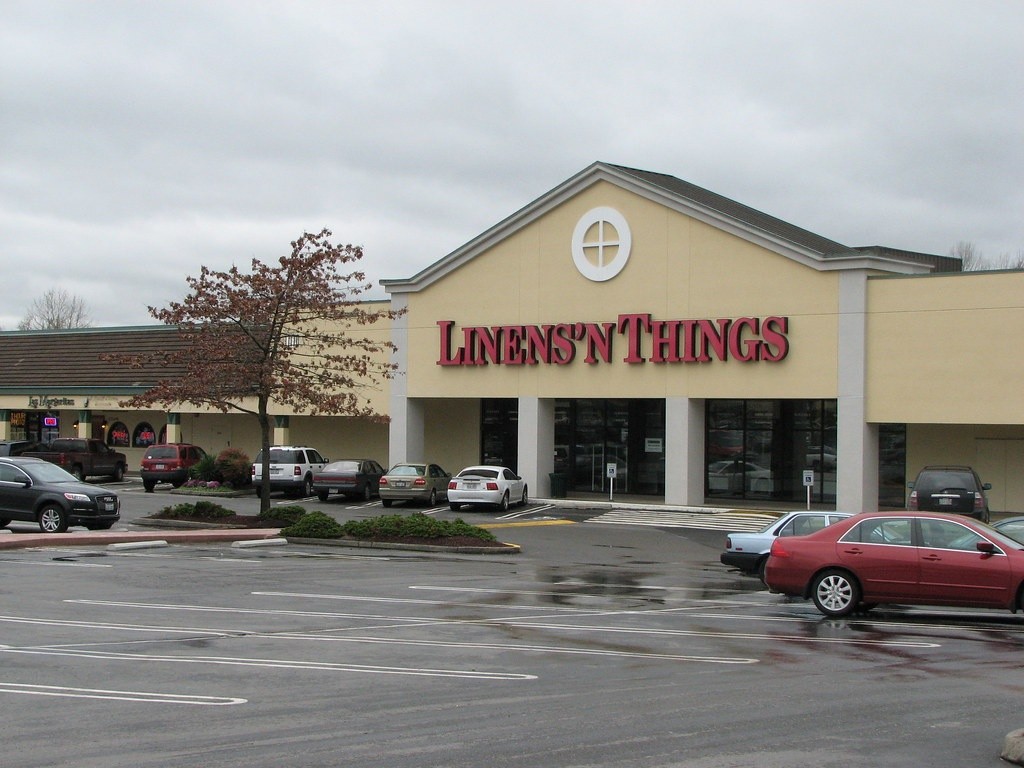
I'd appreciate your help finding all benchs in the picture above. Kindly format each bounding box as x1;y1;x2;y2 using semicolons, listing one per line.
783;528;806;536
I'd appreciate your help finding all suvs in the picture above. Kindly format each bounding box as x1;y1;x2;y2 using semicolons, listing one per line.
907;465;992;525
252;445;329;498
140;442;207;491
0;439;47;457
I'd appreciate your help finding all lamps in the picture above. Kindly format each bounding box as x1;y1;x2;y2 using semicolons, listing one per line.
84;397;88;407
102;420;107;428
73;420;79;429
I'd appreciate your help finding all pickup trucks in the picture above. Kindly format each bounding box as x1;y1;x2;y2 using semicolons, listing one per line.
21;437;128;484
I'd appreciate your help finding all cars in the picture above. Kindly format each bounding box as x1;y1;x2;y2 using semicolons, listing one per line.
950;515;1024;552
553;444;635;484
379;463;453;508
708;460;772;494
313;458;390;502
720;511;930;593
0;455;121;534
708;429;743;458
805;445;837;474
447;466;528;512
763;511;1024;619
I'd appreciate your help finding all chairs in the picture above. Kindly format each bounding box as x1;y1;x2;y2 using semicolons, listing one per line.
931;525;947;546
851;532;888;543
